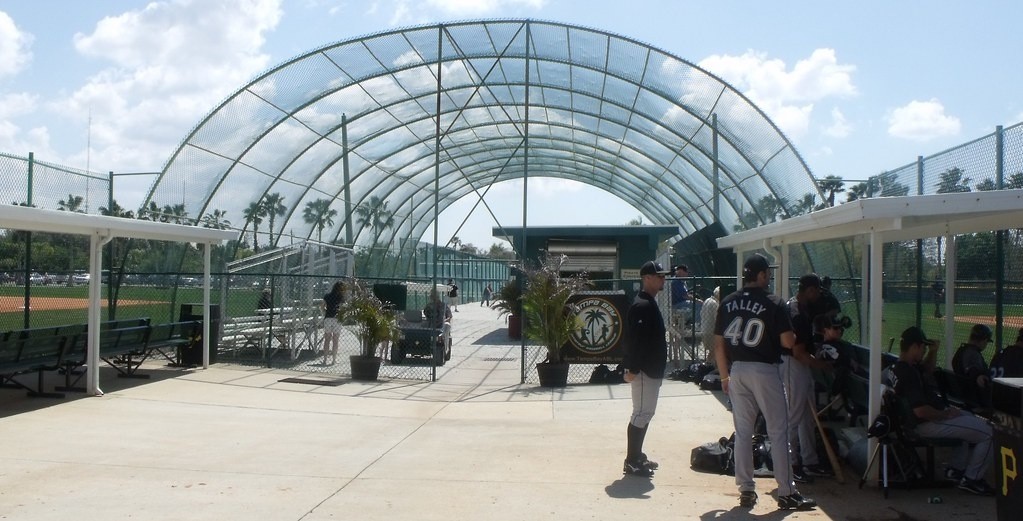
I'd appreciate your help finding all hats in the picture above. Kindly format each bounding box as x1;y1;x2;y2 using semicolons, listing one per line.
820;275;832;289
744;253;779;271
799;274;829;291
972;323;996;344
677;264;691;273
640;262;671;277
901;326;935;345
829;319;843;327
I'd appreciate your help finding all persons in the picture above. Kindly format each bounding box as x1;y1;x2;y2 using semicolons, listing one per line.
258;288;279;322
426;293;452;354
714;255;817;510
881;328;995;495
320;280;347;365
700;286;720;362
779;273;865;482
953;323;994;387
989;328;1023;378
447;279;459;312
672;264;704;322
481;283;493;306
932;275;943;318
624;260;671;476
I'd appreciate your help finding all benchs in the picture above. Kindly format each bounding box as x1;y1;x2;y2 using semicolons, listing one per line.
837;341;993;487
0;317;202;398
216;300;325;363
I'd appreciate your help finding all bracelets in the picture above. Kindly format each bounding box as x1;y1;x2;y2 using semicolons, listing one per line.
721;376;729;381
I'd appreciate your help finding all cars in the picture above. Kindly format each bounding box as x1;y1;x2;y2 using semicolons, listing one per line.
122;275;220;287
101;270;116;284
74;273;91;285
30;272;79;285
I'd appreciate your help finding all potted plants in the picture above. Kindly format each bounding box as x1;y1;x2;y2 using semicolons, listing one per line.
333;279;406;380
515;251;596;388
487;279;522;338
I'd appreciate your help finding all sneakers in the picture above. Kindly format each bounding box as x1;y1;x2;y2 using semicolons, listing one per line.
741;490;758;506
778;492;817;510
819;404;831;418
639;454;659;469
804;465;830;477
958;476;996;496
624;460;654;476
942;465;964;481
792;466;812;482
830;407;853;422
